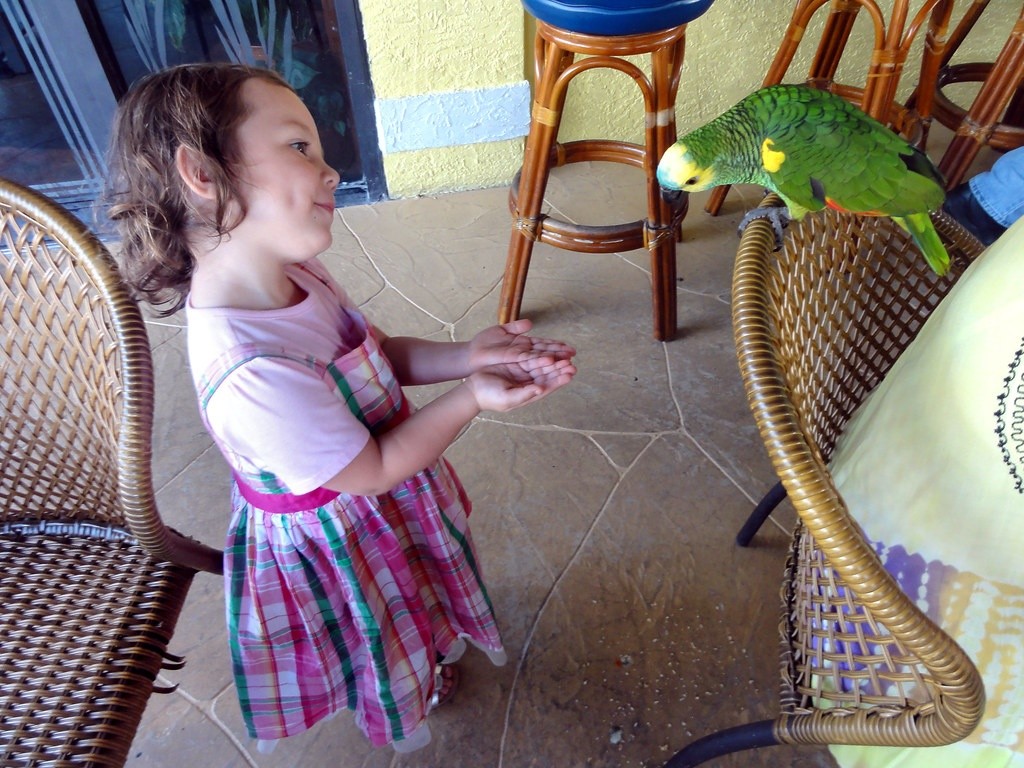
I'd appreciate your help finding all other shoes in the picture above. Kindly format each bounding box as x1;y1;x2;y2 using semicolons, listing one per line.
938;180;1006;257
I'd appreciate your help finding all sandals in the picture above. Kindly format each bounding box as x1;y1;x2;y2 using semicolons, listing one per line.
428;664;459;711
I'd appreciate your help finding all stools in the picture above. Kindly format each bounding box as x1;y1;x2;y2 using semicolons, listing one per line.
498;1;1024;342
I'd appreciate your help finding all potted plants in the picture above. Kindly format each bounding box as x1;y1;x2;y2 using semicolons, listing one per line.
254;41;362;185
147;1;320;62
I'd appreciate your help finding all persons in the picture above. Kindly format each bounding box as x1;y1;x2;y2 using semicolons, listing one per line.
93;61;581;718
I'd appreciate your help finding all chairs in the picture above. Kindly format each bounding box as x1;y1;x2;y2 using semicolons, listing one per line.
664;188;992;768
1;180;225;768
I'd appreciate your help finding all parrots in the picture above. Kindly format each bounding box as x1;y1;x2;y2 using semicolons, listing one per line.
655;84;954;279
